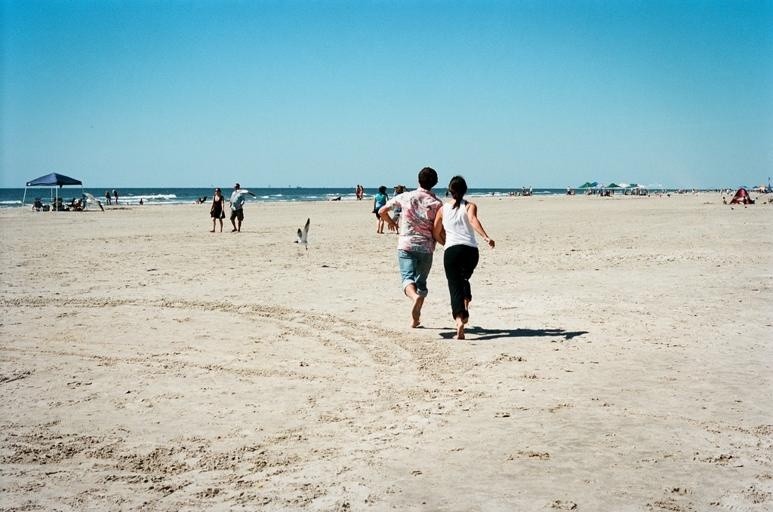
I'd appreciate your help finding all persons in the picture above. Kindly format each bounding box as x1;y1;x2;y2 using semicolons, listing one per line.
209;187;224;233
227;183;244;232
432;176;494;340
377;167;445;329
390;186;405;235
373;186;391;234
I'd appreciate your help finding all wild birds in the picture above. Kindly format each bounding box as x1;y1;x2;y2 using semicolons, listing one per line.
294;218;311;252
